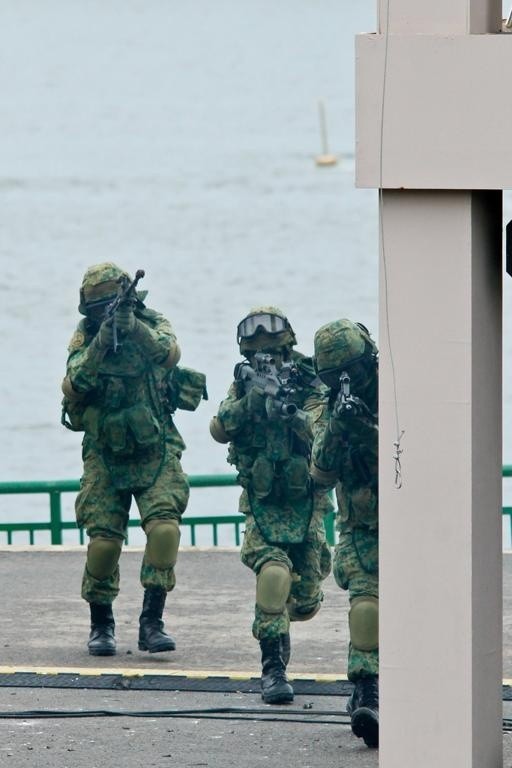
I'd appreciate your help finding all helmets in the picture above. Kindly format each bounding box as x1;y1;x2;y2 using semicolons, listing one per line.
240;306;296;355
314;320;379;395
80;261;137;307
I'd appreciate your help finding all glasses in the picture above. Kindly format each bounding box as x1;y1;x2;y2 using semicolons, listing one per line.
313;342;371;387
237;313;295;346
85;299;116;319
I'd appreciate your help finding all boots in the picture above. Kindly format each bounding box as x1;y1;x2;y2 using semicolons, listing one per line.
88;603;115;655
346;676;379;748
259;633;294;702
138;588;175;652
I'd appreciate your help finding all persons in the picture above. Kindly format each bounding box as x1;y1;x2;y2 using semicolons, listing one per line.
60;261;190;656
305;318;378;749
209;306;331;705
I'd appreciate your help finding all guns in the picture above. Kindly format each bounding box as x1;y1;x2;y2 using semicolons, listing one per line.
334;370;359;418
99;270;145;355
233;352;300;417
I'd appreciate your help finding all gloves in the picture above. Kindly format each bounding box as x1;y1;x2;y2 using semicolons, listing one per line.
114;306;136;333
95;316;114;350
330;406;377;446
241;386;290;422
